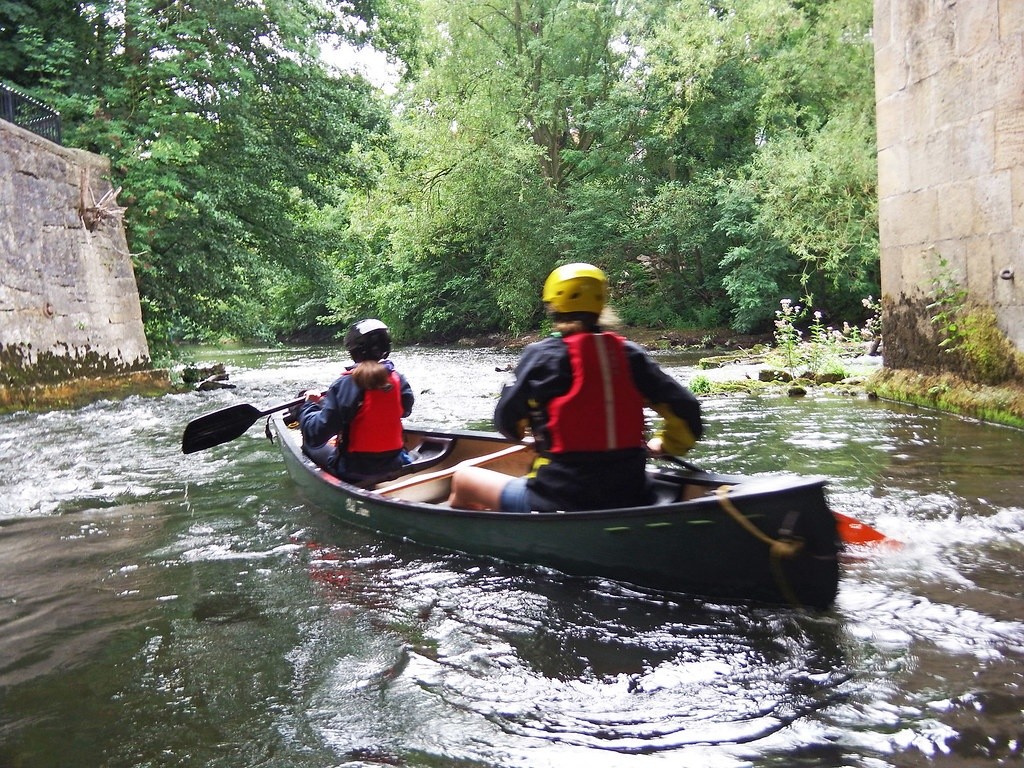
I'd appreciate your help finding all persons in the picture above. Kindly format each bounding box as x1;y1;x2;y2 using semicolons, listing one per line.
299;318;415;483
445;263;704;513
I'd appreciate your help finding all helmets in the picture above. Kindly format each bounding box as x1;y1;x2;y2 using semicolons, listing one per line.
344;318;392;362
542;263;607;325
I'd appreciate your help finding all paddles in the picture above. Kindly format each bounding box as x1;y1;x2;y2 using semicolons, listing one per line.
655;451;912;558
180;386;331;460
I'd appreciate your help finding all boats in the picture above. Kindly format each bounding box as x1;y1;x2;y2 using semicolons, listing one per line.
263;402;844;612
